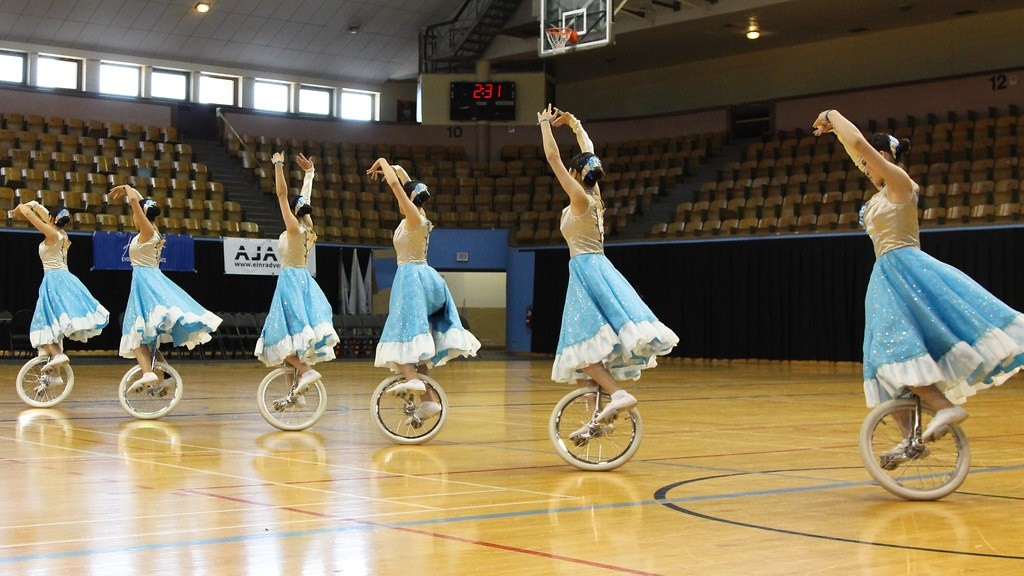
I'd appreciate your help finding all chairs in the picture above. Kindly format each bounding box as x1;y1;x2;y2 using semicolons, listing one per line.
2;309;35;359
119;309;390;360
0;104;1024;244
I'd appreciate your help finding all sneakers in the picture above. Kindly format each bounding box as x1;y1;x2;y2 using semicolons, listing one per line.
128;372;177;396
569;394;637;440
295;371;322;394
921;404;969;443
880;436;930;462
33;376;63;392
385;381;426;395
273;393;306;408
405;402;441;426
40;354;70;371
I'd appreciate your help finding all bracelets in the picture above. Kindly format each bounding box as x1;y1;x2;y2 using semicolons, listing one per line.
536;117;550;125
122;184;127;192
274;160;284;166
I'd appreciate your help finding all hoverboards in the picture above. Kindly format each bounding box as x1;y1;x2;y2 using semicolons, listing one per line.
857;392;971;502
549;383;645;472
370;371;448;446
256;356;329;431
15;350;74;408
119;336;185;419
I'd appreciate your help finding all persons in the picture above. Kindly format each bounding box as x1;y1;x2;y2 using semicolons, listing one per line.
366;157;481;426
812;108;1023;470
252;152;341;411
107;184;224;395
535;103;680;442
7;200;110;393
